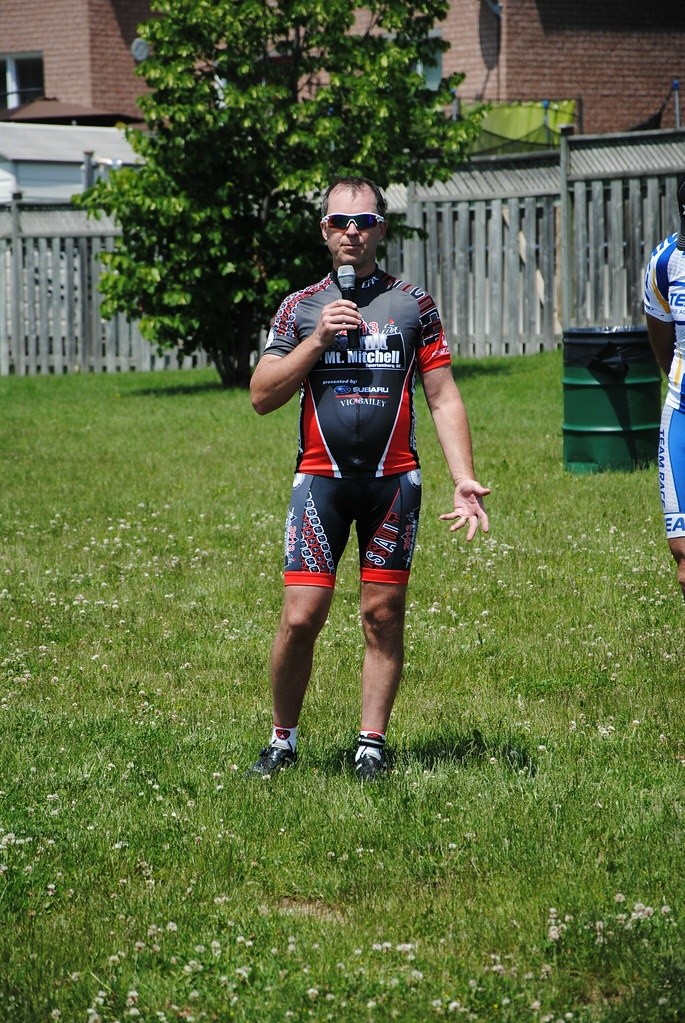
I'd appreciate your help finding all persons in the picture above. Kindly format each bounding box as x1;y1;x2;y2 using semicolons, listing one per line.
641;171;685;599
248;168;492;783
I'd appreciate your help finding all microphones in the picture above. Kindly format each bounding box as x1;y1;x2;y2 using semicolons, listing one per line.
337;264;360;348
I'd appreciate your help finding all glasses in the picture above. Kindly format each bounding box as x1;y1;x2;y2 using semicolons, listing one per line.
322;212;385;228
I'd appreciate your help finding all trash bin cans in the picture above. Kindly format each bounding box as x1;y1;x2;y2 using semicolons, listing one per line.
563;325;663;476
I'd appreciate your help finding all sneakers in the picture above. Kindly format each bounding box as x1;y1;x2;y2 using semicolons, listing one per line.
354;749;390;782
246;746;298;780
